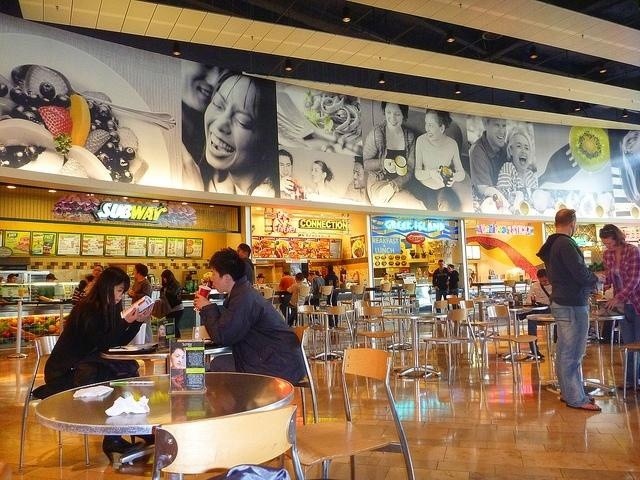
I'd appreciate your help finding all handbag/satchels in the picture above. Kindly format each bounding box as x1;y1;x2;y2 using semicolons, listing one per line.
152;295;172;318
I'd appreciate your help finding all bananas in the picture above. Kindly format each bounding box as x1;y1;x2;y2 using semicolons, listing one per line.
69;93;92;148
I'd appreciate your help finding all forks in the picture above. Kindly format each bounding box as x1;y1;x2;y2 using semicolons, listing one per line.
277;110;363;155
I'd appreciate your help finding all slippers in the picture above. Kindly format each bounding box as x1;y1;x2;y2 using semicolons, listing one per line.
567;403;601;411
560;397;594;403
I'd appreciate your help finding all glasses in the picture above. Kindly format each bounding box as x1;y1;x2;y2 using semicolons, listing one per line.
600;229;617;235
569;209;576;215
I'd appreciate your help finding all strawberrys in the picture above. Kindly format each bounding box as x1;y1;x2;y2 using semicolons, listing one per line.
37;103;72;158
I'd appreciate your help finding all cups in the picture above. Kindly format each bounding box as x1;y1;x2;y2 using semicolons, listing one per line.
393;155;407;176
198;285;211;298
439;165;453;184
134;323;146;345
18;288;25;296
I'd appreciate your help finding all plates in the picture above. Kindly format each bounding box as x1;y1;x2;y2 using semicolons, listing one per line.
351;240;365;258
1;32;171;188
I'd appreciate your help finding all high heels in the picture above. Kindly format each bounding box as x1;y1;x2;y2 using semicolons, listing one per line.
103;437;144;464
131;435;154;446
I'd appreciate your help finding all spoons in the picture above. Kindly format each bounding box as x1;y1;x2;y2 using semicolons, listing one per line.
11;64;177;134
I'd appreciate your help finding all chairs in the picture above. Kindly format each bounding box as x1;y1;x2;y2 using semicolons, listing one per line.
153;405;305;480
19;335;90;470
191;324;210;341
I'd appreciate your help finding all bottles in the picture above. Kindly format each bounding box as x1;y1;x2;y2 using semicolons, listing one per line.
530;292;536;305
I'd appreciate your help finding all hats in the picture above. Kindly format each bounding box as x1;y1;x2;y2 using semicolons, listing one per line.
46;274;58;280
8;274;18;279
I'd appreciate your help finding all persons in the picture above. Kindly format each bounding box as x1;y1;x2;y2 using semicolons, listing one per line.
447;264;459;309
380;273;391;292
536;209;607;411
182;59;230;165
193;247;307;386
1;274;24;305
363;101;428;212
204;70;279;198
526;269;557;360
597;224;640;391
44;264;184;461
496;126;536;206
256;264;338;328
405;109;465;211
469;118;536;200
311;161;332;184
279;149;303;200
432;260;450;320
236;243;256;286
353;156;367;194
37;273;59;301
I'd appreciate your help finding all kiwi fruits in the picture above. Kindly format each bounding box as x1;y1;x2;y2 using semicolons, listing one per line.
569;127;610;173
